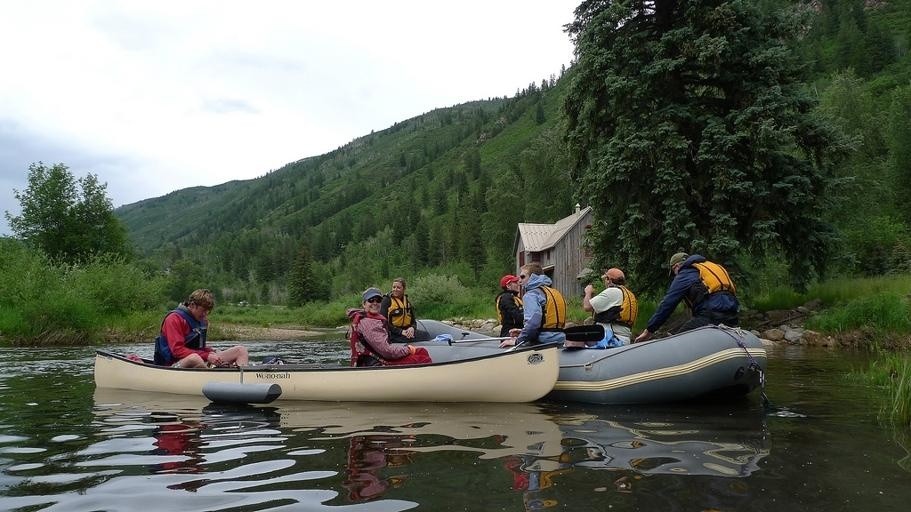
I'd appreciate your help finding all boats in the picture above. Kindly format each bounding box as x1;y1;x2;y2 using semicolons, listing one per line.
404;318;766;409
92;344;560;414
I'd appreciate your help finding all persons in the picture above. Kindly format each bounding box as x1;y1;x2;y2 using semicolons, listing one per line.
633;251;739;345
380;277;430;343
496;262;567;351
345;287;433;368
147;409;210;493
343;426;417;505
581;268;639;349
160;288;248;369
505;443;576;512
494;275;524;342
587;428;664;497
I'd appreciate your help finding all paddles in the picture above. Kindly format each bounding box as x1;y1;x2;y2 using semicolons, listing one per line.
446;325;605;346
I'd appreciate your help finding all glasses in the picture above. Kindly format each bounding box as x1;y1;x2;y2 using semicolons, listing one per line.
368;298;382;303
521;275;529;279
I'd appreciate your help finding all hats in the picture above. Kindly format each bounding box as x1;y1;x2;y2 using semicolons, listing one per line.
601;268;625;280
363;287;383;301
669;252;690;277
500;275;519;286
